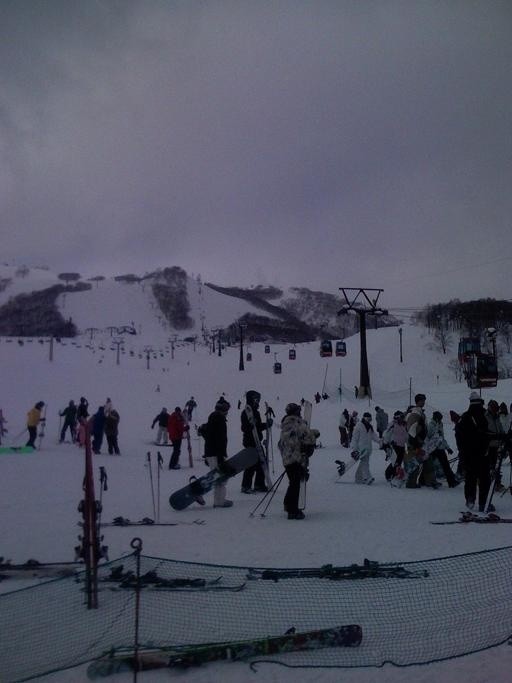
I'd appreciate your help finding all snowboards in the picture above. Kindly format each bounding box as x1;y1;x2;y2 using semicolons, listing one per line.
390;438;443;488
169;447;258;509
332;448;367;482
0;446;34;454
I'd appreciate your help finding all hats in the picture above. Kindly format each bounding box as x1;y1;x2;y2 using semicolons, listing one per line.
216;398;229;411
286;403;300;414
468;392;483;405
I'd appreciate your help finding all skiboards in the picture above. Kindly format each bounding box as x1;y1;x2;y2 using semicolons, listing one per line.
246;568;430;579
0;558;133;581
246;401;275;490
76;518;205;525
86;624;362;680
298;399;312;510
81;576;246;592
429;516;511;524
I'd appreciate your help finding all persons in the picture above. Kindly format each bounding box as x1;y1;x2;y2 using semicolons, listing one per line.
26;399;47;450
278;402;320;520
238;390;273;493
450;392;512;520
338;395;459;488
204;396;233;507
56;395;121;456
353;386;359;398
301;391;329;405
152;397;198;469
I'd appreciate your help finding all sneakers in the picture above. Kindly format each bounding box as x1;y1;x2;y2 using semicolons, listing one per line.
405;479;460;489
242;488;256;494
197;495;206;504
213;500;234;507
